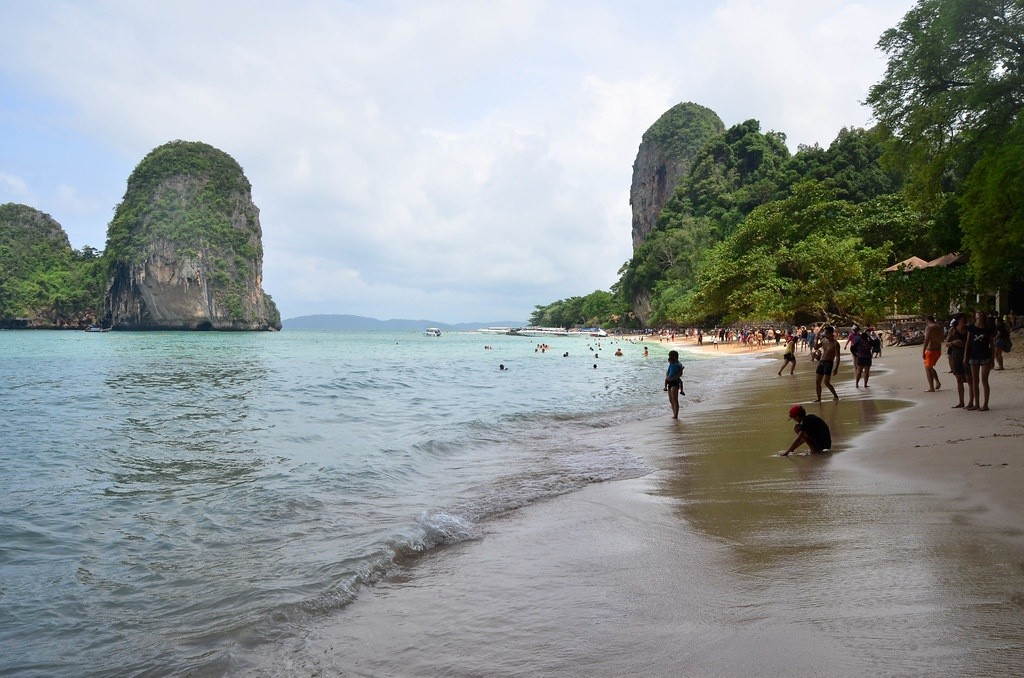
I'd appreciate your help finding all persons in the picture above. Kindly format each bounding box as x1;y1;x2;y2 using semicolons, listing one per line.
663;350;686;420
485;328;703;371
711;322;884;388
814;326;840;402
946;309;1017;412
892;323;925;347
922;315;943;392
780;404;831;458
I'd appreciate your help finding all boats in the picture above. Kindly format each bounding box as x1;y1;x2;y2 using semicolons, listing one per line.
424;328;442;337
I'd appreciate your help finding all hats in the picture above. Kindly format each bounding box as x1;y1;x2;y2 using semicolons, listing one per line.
851;325;857;329
789;406;805;421
951;312;972;319
